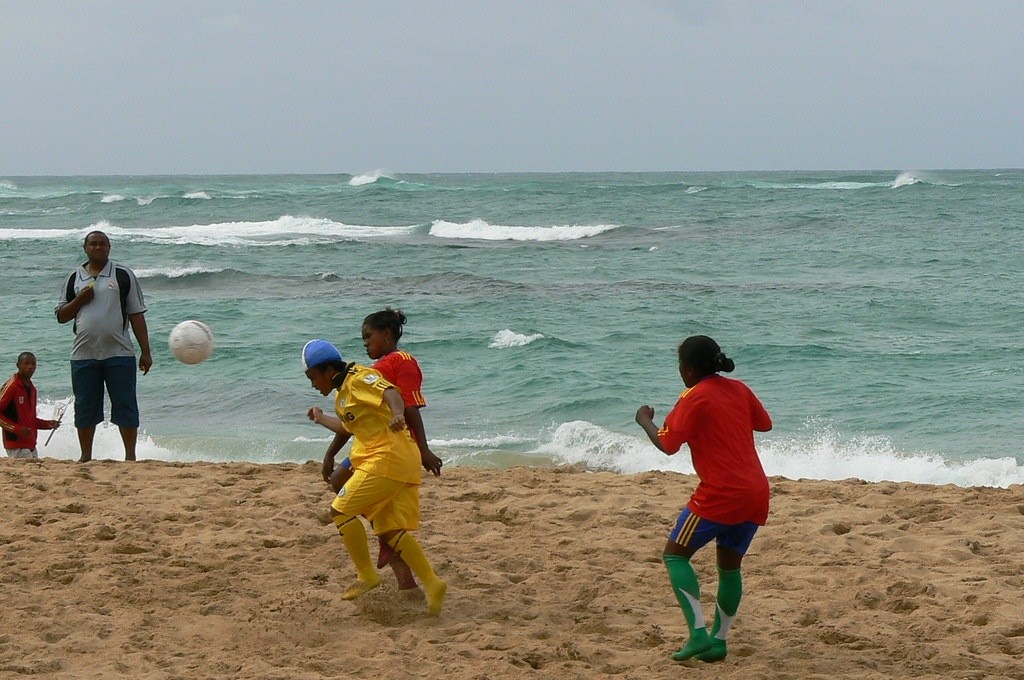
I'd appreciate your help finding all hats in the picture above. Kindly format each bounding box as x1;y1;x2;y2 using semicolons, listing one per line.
302;339;342;372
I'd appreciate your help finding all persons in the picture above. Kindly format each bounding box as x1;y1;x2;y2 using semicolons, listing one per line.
302;339;448;621
0;352;62;457
636;335;772;661
321;308;443;589
54;231;154;461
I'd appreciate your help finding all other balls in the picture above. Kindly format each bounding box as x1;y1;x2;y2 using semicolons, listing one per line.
168;318;214;365
